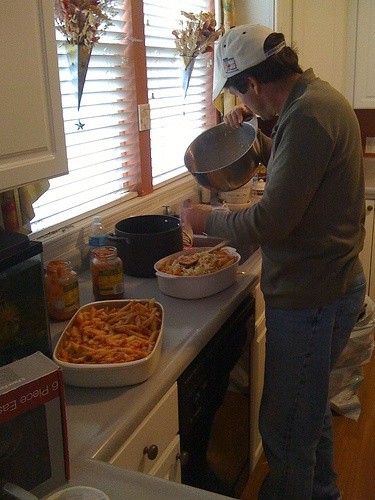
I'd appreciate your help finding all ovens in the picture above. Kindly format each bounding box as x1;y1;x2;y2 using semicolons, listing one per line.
173;294;258;499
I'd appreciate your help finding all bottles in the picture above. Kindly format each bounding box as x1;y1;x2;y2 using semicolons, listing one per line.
89;216;105;248
44;259;80;320
90;247;125;299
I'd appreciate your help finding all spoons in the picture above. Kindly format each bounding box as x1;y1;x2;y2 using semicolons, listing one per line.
179;237;229;264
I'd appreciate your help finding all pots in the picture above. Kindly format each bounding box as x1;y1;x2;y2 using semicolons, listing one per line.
107;213;183;278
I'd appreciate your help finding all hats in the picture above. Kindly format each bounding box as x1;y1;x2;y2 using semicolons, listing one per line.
211;23;287;101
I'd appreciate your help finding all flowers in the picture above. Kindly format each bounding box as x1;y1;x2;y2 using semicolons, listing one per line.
53;0;119;50
171;9;223;58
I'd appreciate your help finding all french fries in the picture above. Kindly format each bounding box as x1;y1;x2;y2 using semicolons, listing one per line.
58;297;162;364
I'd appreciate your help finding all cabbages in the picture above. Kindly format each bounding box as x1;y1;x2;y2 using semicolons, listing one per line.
166;251;218;276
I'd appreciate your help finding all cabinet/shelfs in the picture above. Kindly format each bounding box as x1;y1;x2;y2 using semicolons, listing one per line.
110;381;190;483
0;0;69;193
292;0;375;109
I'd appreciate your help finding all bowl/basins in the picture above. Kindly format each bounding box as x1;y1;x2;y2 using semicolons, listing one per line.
154;245;241;299
223;200;252;212
185;120;261;193
224;188;249;204
43;486;110;500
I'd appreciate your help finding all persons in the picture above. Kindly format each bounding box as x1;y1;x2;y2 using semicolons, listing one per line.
185;24;367;500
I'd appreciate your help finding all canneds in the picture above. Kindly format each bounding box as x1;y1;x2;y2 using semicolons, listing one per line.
45;260;80;321
91;246;125;301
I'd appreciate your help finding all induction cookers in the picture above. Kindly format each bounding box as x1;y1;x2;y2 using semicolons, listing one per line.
117;203;262;272
